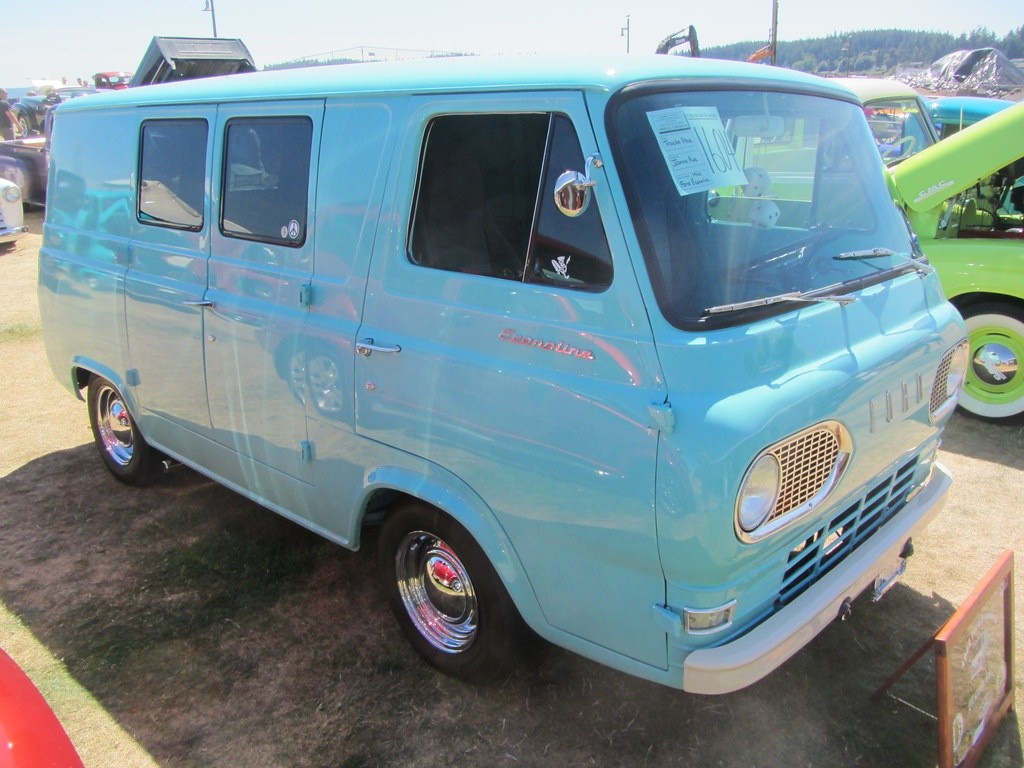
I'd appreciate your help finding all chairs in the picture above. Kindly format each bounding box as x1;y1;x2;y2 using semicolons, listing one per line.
619;136;710;321
412;134;524;283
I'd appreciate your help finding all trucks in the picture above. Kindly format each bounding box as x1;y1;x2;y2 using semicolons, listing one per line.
0;104;58;211
94;72;134;90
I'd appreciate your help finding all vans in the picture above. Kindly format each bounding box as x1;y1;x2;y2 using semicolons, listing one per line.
36;55;970;695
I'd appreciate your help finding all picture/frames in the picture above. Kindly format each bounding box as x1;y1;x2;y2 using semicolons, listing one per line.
934;548;1015;768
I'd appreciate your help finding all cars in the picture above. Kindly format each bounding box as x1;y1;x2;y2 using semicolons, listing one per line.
708;79;1024;424
0;178;29;244
900;96;1024;216
